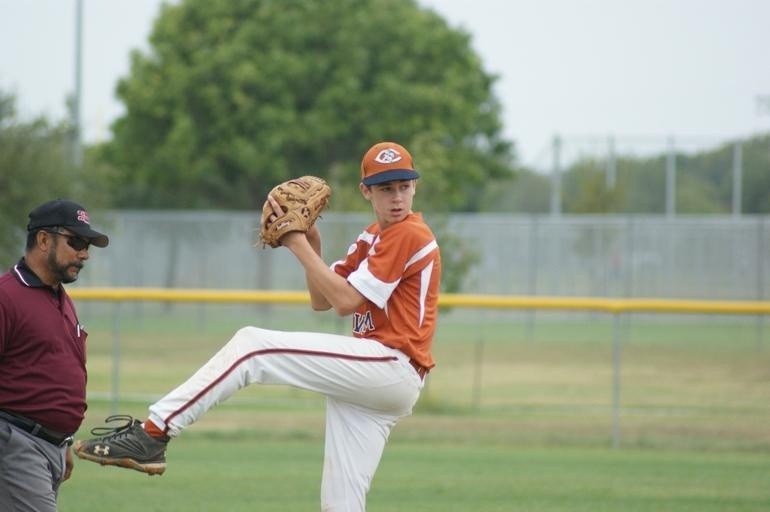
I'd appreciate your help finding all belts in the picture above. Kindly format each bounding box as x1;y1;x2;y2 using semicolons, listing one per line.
0;410;74;447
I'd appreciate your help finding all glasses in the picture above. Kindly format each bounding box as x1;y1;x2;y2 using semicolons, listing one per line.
43;229;89;251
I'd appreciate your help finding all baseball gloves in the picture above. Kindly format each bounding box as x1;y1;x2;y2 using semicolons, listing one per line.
258;174;331;249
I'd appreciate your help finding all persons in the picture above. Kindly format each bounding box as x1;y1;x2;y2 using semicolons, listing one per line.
73;142;445;512
1;200;109;512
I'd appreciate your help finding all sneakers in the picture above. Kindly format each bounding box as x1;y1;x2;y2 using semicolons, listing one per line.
73;419;167;474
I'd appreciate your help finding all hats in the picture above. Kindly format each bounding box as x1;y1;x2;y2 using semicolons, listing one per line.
27;198;110;248
360;141;420;185
